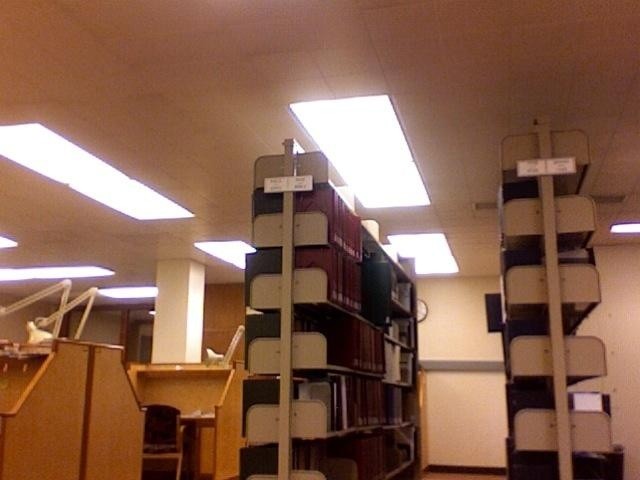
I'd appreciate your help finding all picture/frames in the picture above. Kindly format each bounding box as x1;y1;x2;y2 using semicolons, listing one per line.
127;360;243;479
0;333;145;480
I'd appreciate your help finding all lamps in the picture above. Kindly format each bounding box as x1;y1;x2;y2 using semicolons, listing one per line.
143;405;185;480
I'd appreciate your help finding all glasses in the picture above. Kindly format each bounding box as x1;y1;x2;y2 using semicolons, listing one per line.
25;287;99;345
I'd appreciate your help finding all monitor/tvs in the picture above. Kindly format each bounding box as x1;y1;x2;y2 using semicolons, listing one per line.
296;191;418;478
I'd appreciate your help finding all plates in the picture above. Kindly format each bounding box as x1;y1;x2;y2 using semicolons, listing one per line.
493;121;626;480
246;150;422;480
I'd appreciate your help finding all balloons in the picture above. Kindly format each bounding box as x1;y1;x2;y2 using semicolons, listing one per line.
416;298;428;324
